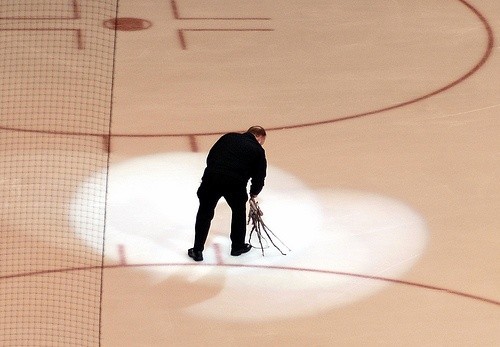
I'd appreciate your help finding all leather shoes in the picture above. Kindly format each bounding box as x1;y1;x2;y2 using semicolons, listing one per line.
188;248;203;261
230;243;251;256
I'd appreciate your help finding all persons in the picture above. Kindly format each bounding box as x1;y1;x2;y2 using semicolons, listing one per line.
186;125;268;263
